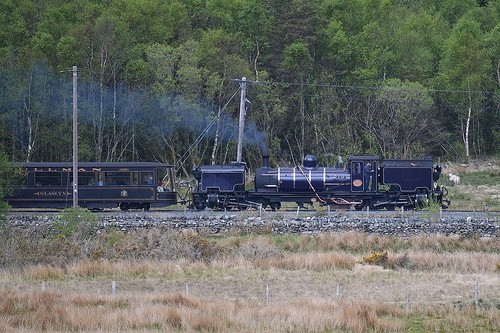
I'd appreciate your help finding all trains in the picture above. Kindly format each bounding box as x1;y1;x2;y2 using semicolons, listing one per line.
1;154;451;212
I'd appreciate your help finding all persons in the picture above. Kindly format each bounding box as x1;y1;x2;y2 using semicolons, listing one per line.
87;175;154;186
364;163;375;192
157;177;165;192
36;180;42;186
